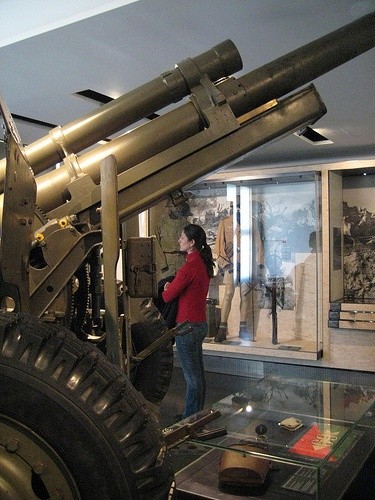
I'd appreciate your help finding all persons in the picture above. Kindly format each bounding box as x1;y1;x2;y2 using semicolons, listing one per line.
162;224;216;422
214;197;261;343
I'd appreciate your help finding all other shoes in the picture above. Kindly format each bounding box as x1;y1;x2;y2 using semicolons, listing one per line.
175;415;183;421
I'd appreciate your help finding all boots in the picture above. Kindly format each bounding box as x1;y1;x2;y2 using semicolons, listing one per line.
215;322;227;342
240;320;251;341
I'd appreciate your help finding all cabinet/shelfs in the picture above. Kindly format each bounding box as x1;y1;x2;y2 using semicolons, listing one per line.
150;373;375;500
102;154;375;392
